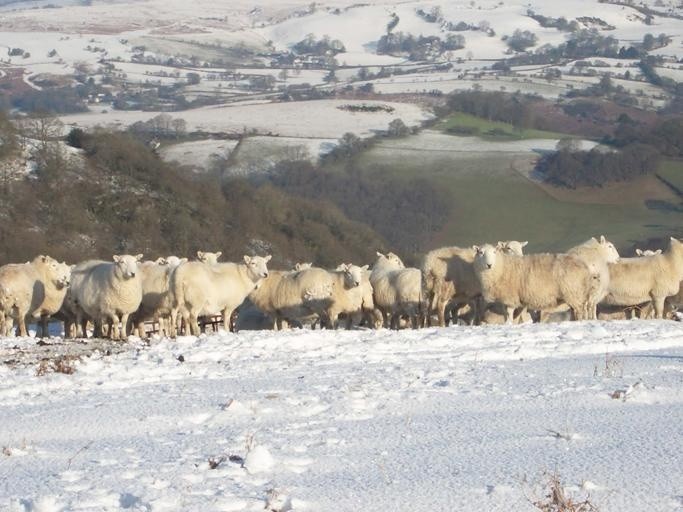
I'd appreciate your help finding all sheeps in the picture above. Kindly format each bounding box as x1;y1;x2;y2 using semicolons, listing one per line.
0;232;683;342
